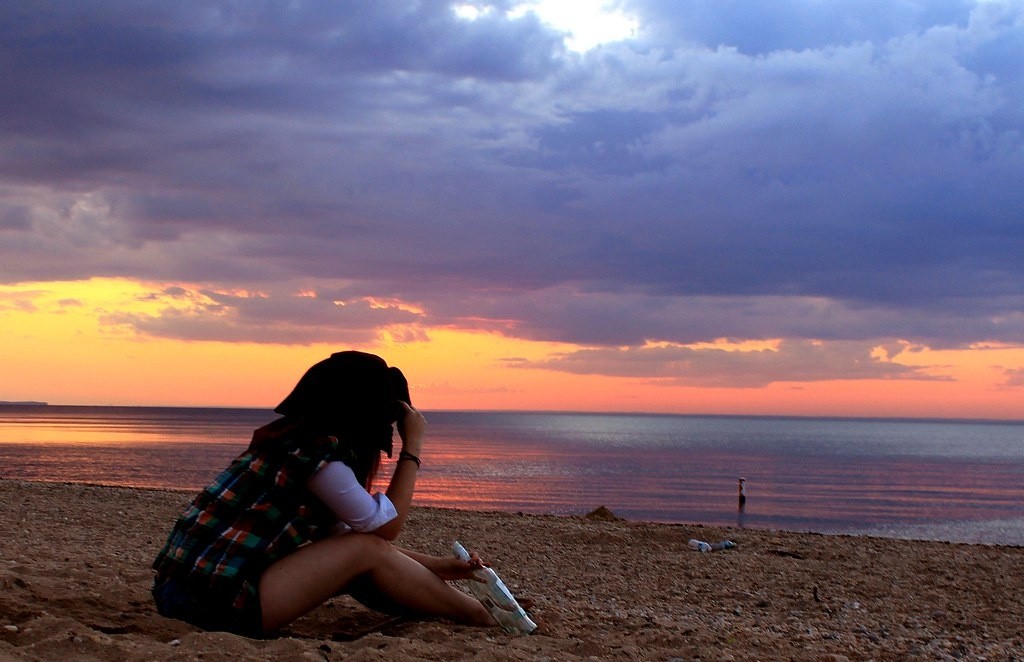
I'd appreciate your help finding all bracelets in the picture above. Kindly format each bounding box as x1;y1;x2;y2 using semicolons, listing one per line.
397;451;421;469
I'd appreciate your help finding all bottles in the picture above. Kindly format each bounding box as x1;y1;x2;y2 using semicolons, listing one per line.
688;538;712;552
448;539;538;639
710;541;739;549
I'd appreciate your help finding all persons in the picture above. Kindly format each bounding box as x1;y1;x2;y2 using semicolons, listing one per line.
738;477;746;505
152;350;534;637
